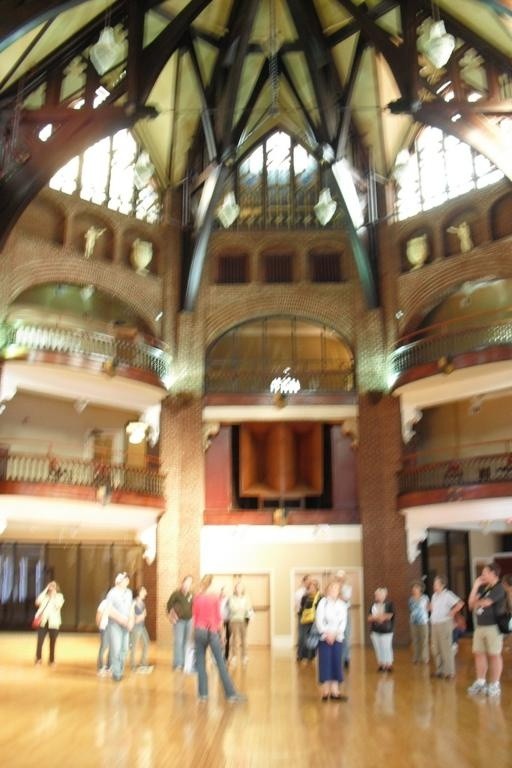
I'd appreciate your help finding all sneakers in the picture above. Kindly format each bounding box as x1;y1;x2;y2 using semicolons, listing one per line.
483;683;501;697
414;659;430;665
172;664;197;673
97;666;153;680
330;694;349;701
226;693;248;703
322;694;330;701
35;659;57;668
200;694;210;703
467;680;483;694
378;665;395;672
212;656;249;669
433;672;456;678
296;660;352;669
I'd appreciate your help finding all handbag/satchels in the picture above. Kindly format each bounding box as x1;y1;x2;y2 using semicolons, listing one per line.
31;615;42;630
492;580;512;633
300;604;316;625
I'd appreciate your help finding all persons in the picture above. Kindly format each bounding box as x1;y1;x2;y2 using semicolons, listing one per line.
165;573;195;674
105;569;135;683
129;583;154;680
191;572;254;705
293;565;355;704
428;573;465;684
95;583;113;675
450;562;512;697
82;225;109;261
406;578;432;664
366;584;398;674
32;579;65;669
